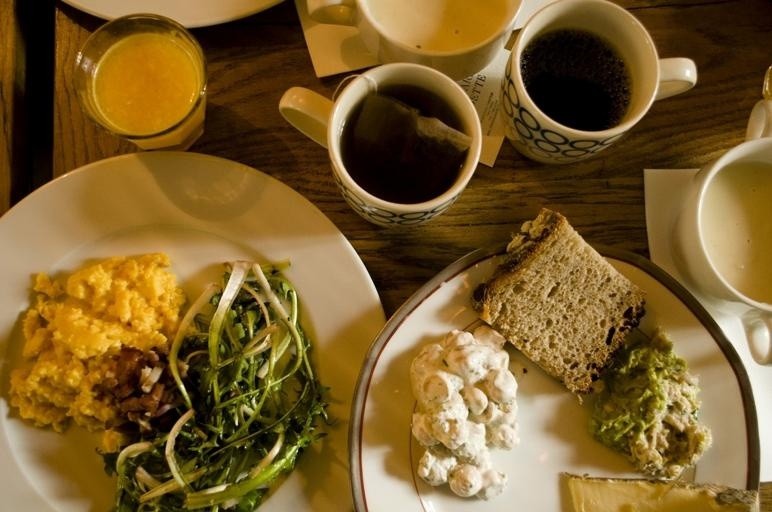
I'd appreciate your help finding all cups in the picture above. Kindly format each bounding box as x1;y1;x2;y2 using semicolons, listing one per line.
498;0;697;165
71;12;209;152
306;0;525;83
675;99;772;369
278;63;483;230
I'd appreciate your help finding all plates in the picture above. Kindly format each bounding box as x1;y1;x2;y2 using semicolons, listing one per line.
0;151;389;512
347;240;760;512
61;0;286;29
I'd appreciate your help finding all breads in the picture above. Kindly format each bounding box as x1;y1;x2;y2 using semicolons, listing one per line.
472;210;648;394
